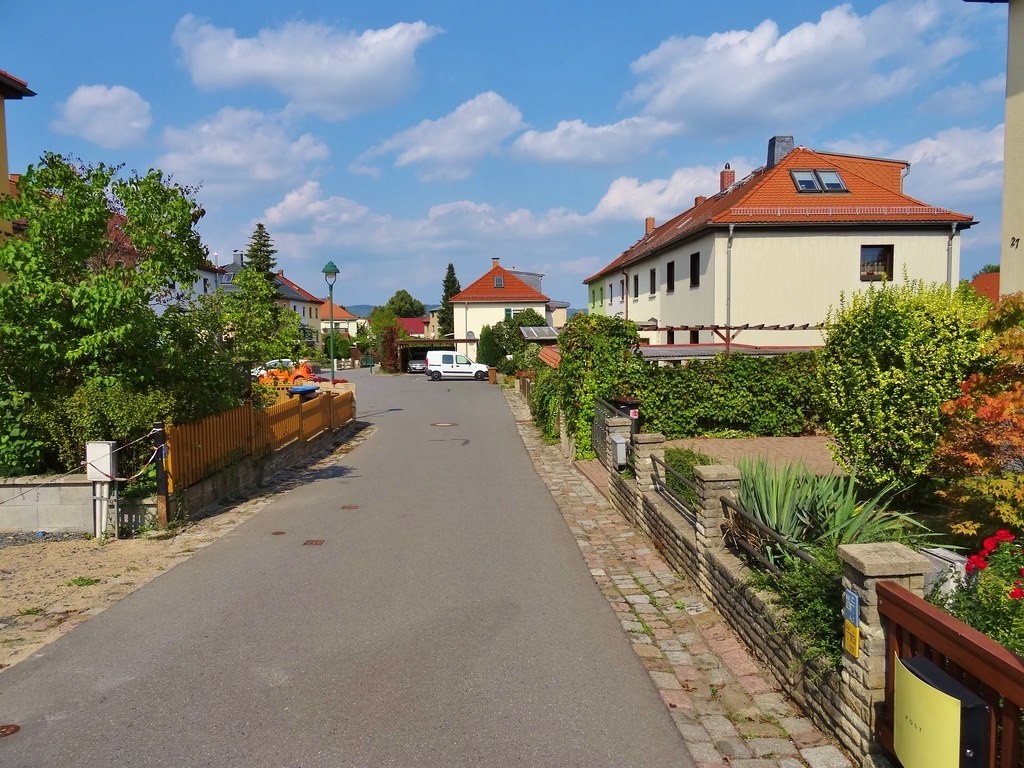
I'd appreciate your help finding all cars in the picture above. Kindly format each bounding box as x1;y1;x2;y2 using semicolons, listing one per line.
408;352;425;374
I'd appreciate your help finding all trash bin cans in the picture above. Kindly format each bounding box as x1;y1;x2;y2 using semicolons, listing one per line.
362;355;374;366
288;384;320;403
604;395;641;455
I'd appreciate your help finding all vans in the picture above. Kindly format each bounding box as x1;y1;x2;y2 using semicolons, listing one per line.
251;358;315;383
425;350;489;382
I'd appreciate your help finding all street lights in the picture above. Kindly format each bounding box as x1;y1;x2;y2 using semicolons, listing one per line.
324;261;341;396
